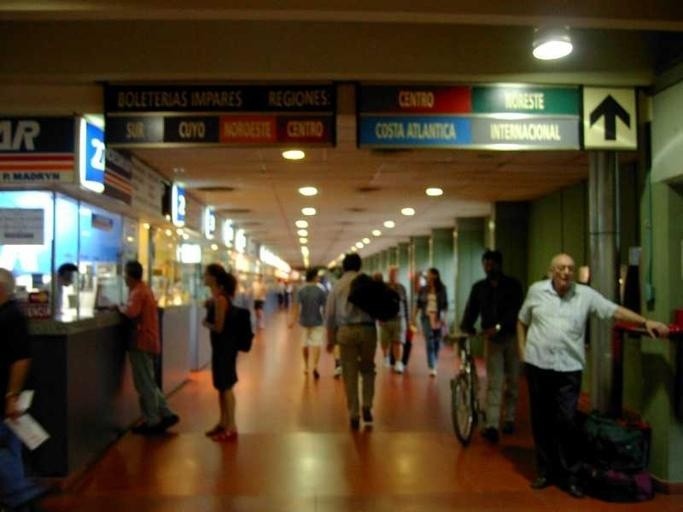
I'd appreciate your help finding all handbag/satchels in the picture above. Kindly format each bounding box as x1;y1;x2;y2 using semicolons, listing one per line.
346;272;400;322
572;405;654;502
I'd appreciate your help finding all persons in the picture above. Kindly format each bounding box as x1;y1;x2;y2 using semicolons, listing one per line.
246;273;267;331
199;264;254;443
411;267;448;378
287;267;333;379
517;254;671;498
409;270;426;321
460;247;524;443
39;261;78;323
107;261;180;438
273;277;292;311
372;270;384;283
324;253;377;432
378;266;411;373
1;269;42;511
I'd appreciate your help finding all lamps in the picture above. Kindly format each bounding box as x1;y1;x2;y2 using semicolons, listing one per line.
532;21;574;60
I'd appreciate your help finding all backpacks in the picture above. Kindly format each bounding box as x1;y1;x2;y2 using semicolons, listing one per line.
219;293;256;353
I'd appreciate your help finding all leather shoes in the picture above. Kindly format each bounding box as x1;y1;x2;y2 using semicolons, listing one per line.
531;473;552;489
558;477;583;500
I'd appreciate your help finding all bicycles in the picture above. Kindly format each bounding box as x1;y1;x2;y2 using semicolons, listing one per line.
439;328;487;446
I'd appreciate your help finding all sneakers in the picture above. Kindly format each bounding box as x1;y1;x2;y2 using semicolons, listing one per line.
311;369;320;380
132;419;166;435
361;405;373;424
503;421;515;435
206;423;222;435
381;357;391;371
348;417;360;428
332;367;343;382
476;428;500;444
429;365;439;379
394;360;406;375
155;415;179;429
212;428;240;443
